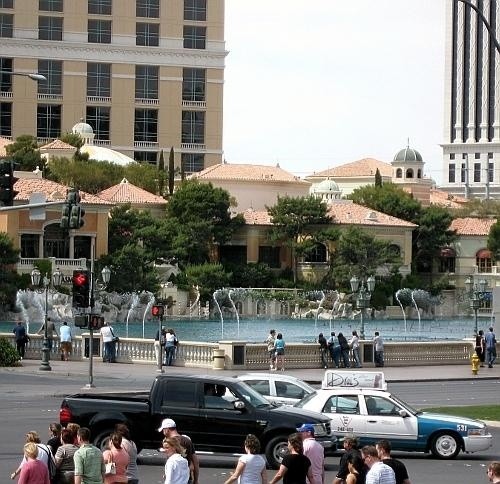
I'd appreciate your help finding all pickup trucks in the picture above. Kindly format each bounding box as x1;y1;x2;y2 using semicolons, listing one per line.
55;372;335;471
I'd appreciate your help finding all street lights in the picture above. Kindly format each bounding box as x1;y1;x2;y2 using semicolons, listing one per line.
463;274;489;339
349;273;378;339
81;266;112;390
29;266;66;372
0;69;49;82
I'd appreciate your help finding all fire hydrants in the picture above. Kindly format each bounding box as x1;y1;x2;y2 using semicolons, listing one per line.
470;351;480;375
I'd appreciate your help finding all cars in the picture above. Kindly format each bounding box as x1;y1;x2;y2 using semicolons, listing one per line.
277;367;496;463
220;372;319;408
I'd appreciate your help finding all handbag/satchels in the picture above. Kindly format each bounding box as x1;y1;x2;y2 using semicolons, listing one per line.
174;339;178;345
101;463;116;475
112;336;119;343
328;343;334;350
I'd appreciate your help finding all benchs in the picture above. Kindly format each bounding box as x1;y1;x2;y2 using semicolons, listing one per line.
479;308;492;313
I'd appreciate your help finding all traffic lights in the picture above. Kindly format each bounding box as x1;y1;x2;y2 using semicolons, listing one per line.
71;269;90;308
61;190;86;230
91;314;106;331
151;305;165;317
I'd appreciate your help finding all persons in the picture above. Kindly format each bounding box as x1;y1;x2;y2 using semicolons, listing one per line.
204;385;217;407
215;385;236;404
331;438;410;484
318;333;328;369
223;424;325;484
9;422;140;484
158;418;200;484
484;326;497;369
153;326;166;370
37;317;58;351
100;321;116;363
12;322;26;360
327;332;342;368
266;329;277;371
486;462;500;484
474;330;486;367
164;328;178;366
59;322;72;361
338;332;350;368
373;332;385;368
348;330;362;368
274;333;286;372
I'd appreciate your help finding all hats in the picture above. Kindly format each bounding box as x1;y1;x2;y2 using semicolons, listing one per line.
158;419;177;432
339;433;359;441
296;424;314;432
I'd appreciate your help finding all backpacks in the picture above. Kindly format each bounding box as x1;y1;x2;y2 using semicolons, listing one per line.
37;445;56;477
